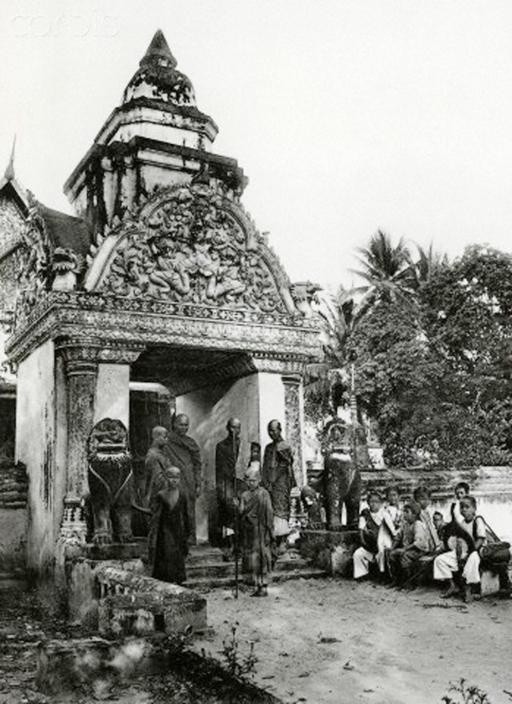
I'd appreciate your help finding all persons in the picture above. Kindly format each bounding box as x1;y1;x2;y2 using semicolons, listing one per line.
214;416;251;545
144;464;198;587
261;417;297;555
162;412;203;548
231;465;275;597
353;480;488;605
247;440;263;488
140;425;177;571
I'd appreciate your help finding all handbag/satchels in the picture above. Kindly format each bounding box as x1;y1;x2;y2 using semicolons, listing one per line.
481;542;511;573
